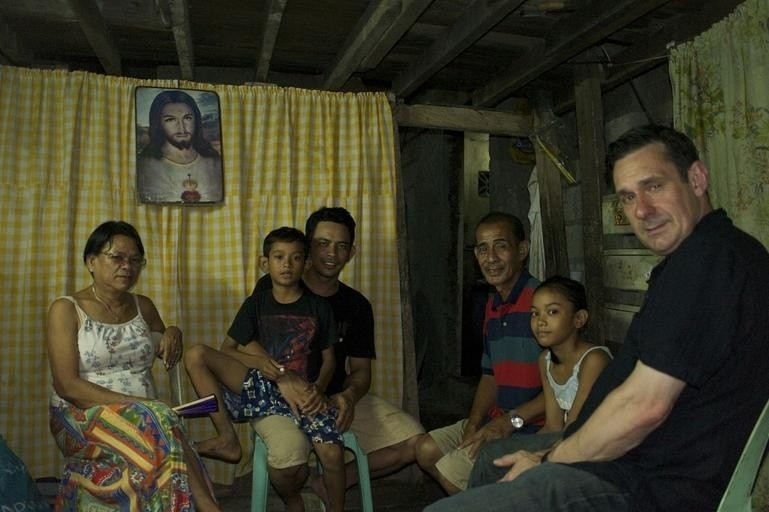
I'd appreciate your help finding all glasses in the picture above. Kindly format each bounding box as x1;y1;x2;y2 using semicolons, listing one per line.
102;251;147;267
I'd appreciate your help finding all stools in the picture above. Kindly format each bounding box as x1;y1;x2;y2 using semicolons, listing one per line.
252;432;373;512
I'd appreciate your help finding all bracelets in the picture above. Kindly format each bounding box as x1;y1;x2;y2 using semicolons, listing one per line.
540;449;551;464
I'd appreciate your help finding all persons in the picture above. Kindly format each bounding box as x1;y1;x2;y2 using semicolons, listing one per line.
218;205;429;511
417;122;769;512
41;217;226;511
413;209;551;498
133;91;224;203
528;275;617;437
179;225;349;511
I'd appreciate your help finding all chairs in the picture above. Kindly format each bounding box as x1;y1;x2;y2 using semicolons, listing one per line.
712;400;768;512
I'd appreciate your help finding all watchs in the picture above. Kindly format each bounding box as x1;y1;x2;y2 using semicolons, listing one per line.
508;409;525;433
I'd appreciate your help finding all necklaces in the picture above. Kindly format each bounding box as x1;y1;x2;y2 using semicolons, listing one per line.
91;284;129;324
